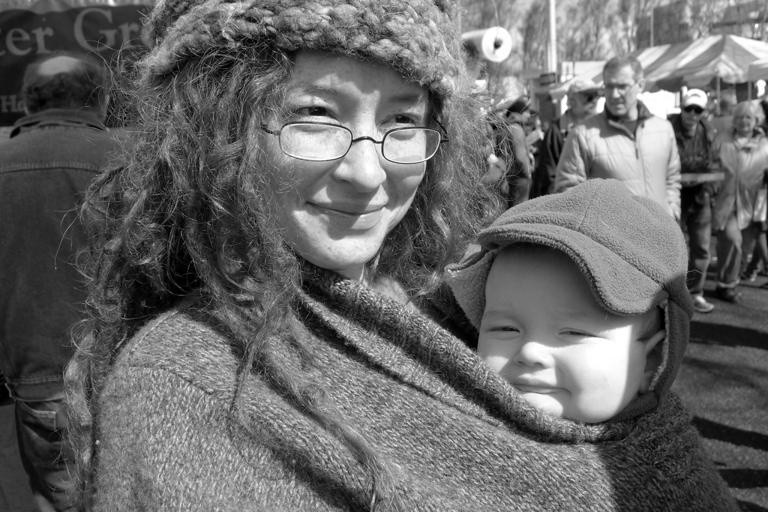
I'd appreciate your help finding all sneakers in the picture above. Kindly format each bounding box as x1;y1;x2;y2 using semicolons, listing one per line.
740;272;755;282
690;293;714;313
712;283;743;303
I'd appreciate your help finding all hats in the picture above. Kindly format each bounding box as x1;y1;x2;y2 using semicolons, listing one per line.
136;0;459;100
563;75;605;96
505;99;529;117
681;89;708;110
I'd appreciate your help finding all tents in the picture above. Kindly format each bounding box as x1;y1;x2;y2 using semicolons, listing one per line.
534;32;768;114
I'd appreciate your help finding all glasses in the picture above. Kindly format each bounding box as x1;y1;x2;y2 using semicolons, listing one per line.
255;111;449;165
684;105;705;114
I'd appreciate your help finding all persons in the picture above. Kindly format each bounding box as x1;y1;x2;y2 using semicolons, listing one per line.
0;49;129;512
667;87;717;314
712;101;734;132
742;98;768;291
707;100;768;303
83;0;741;512
505;100;534;207
554;54;682;222
541;77;598;193
